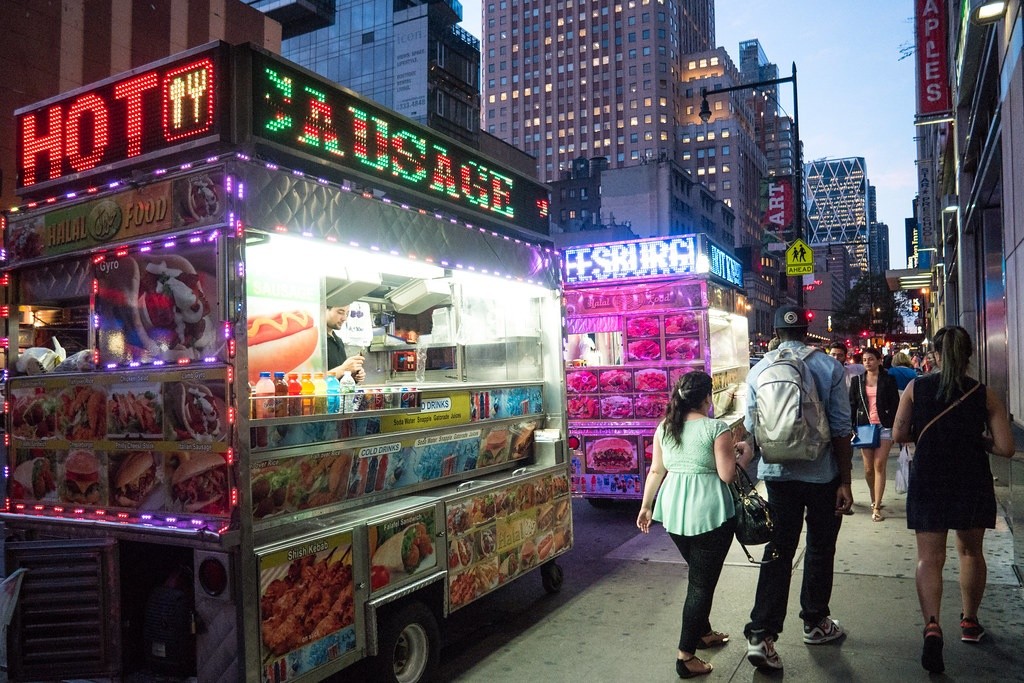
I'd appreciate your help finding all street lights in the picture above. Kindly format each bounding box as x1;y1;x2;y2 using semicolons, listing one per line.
824;241;874;331
698;62;802;334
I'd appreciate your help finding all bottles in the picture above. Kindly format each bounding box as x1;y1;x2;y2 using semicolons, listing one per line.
473;391;489;419
356;387;420;411
255;372;276;418
570;473;640;493
274;372;290;417
299;372;316;415
286;373;303;416
312;372;328;415
340;370;356;414
324;371;340;414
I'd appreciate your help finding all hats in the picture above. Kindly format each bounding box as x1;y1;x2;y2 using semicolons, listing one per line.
774;304;809;329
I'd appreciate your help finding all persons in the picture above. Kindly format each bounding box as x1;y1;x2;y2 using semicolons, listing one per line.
325;304;366;386
637;304;1016;678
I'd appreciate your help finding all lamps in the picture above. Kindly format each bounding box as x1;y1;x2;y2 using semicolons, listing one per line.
969;0;1009;27
941;194;958;213
934;257;944;267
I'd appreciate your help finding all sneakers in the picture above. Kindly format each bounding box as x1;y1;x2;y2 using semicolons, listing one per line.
921;615;945;673
960;612;985;641
802;617;843;643
747;628;783;670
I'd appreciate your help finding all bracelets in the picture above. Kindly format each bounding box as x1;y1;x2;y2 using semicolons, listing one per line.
840;473;851;484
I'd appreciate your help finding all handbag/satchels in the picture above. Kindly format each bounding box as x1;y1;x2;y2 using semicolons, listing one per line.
732;462;777;545
850;424;880;450
894;443;912;494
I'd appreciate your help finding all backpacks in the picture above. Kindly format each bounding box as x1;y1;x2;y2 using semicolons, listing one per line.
753;346;830;463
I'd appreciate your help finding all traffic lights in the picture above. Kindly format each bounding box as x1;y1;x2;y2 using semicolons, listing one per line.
808;312;813;322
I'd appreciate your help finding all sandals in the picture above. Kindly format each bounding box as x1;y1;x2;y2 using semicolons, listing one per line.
871;501;882;509
872;507;882;522
676;656;713;678
696;629;729;649
